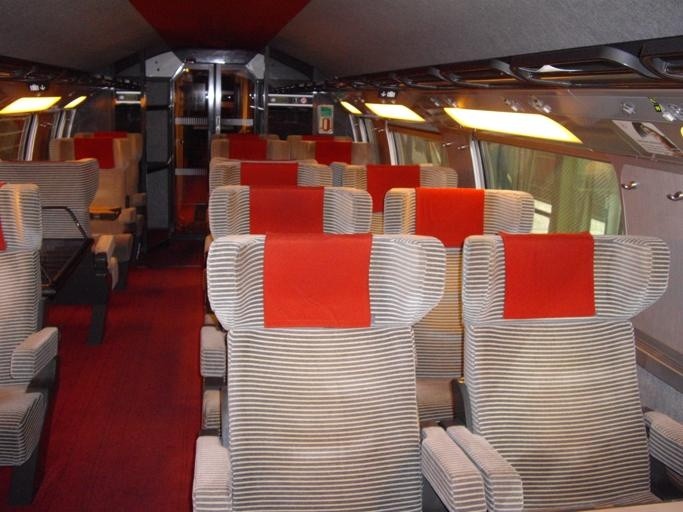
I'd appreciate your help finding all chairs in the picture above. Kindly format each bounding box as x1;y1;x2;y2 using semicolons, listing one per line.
0;131;147;511
194;132;682;510
